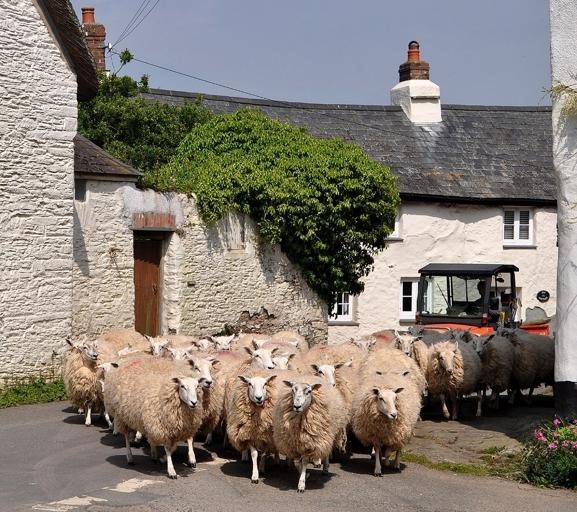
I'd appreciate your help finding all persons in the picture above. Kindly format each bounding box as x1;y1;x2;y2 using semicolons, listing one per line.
470;280;493;310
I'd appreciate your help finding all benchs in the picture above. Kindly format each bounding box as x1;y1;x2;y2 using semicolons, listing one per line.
448;300;512;324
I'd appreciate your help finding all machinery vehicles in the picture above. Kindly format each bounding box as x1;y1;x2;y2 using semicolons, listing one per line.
416;260;553;340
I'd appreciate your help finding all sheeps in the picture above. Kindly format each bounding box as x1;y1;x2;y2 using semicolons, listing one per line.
63;326;555;492
